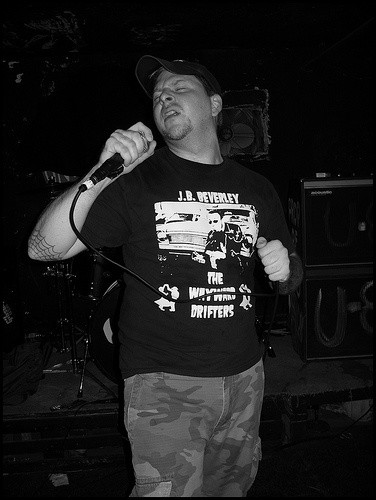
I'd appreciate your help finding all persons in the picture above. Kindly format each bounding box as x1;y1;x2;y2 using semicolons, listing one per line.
28;56;304;498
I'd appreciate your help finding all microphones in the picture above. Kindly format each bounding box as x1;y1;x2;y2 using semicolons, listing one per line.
79;133;149;191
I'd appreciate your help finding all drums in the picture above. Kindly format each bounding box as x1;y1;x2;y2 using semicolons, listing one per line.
92;280;125;384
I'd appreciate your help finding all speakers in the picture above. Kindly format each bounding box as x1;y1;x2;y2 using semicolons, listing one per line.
288;276;376;362
300;177;376;269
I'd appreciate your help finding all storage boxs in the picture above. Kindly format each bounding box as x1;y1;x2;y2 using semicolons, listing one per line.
289;174;376;362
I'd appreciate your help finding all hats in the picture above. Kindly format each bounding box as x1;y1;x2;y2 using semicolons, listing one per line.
135;54;221;99
